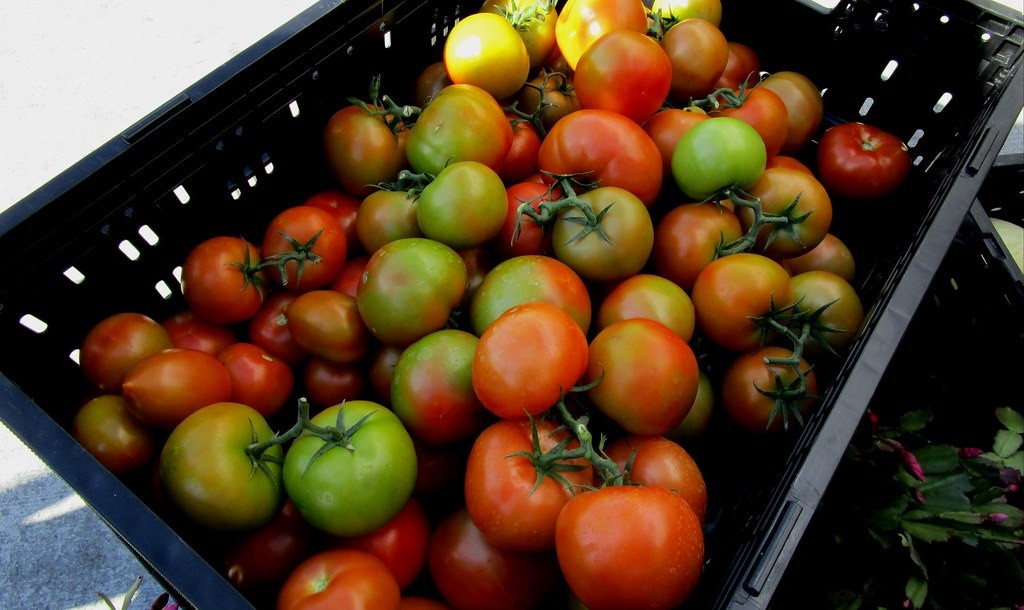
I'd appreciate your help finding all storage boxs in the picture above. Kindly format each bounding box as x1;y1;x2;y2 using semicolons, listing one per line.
0;0;1024;610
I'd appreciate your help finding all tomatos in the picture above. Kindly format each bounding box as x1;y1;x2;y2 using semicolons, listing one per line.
75;0;909;610
